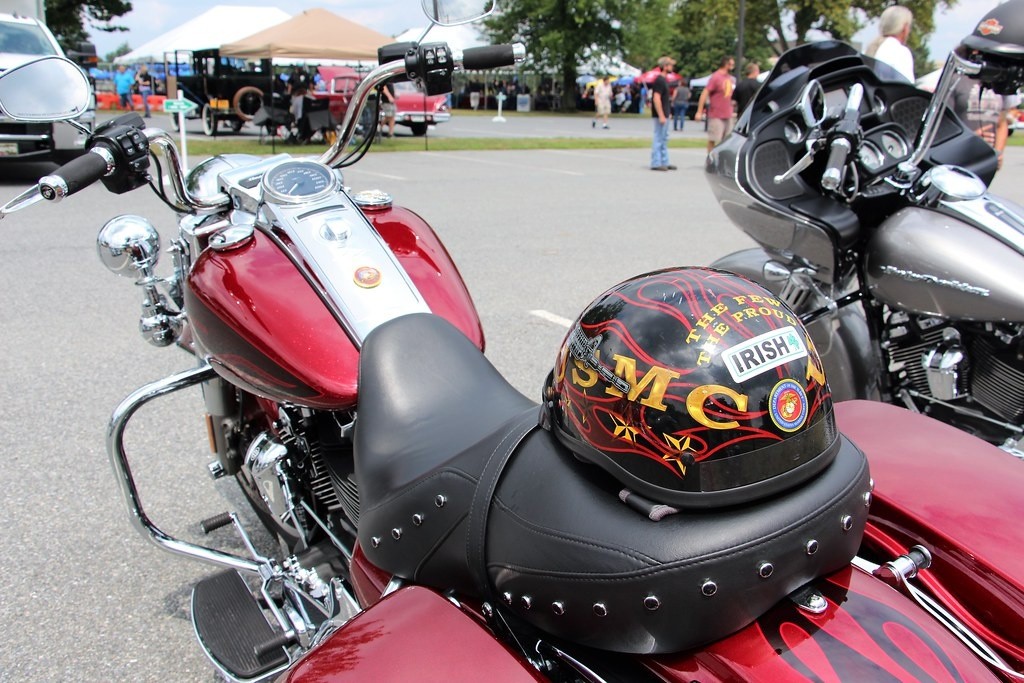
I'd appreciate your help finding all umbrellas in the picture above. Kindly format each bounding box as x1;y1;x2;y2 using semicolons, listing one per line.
575;65;681;87
690;71;736;89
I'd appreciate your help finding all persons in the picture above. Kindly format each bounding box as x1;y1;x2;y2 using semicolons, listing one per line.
593;77;613;129
650;57;677;172
113;64;151;119
274;74;284;87
672;79;691;131
732;64;761;117
621;83;645;112
286;66;310;120
694;56;737;157
967;83;1021;169
381;83;395;138
470;79;561;109
865;6;914;83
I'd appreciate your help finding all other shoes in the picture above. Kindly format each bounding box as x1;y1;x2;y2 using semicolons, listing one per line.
652;163;678;172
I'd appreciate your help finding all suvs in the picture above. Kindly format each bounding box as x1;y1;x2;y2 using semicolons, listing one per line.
162;48;278;137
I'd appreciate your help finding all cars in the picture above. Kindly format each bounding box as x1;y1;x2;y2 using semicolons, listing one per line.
1;14;95;168
313;70;451;137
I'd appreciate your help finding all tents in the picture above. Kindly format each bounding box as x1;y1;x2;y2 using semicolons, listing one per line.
114;5;398;63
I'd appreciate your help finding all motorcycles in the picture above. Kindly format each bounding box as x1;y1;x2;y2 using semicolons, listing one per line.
697;0;1021;448
1;1;1023;682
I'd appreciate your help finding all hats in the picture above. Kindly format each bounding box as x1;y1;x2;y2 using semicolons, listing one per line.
658;57;676;68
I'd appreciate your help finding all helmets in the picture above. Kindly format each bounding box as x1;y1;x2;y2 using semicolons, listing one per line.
961;1;1024;59
538;265;844;516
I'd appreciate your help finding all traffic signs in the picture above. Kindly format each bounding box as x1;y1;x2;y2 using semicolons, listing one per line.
163;99;198;114
